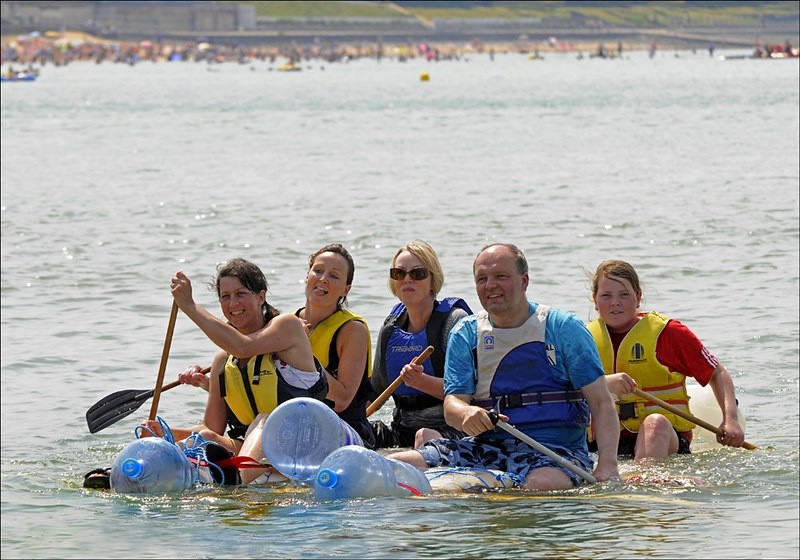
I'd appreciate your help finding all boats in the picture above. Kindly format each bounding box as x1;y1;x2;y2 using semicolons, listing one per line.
206;458;702;502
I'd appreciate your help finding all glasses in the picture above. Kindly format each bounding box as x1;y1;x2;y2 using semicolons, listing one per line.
389;268;430;280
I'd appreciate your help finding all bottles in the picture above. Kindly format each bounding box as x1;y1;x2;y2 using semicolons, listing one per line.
109;437;199;494
313;444;433;502
262;397;365;489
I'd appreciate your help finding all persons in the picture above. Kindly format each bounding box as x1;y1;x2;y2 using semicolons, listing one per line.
584;259;745;459
384;243;620;491
83;244;371;490
365;240;474;450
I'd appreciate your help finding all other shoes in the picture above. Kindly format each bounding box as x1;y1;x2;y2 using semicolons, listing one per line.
84;468;110;489
206;443;242;488
